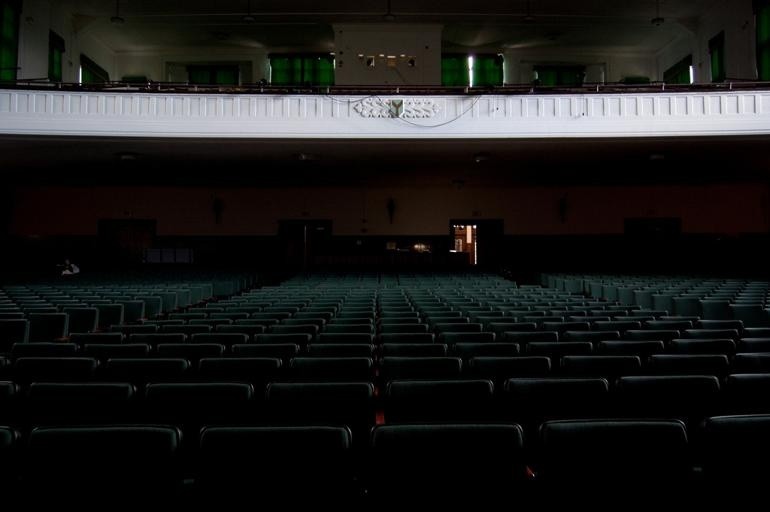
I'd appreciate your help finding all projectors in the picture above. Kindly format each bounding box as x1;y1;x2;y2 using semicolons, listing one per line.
381;14;395;23
649;17;665;25
25;16;34;24
109;16;126;25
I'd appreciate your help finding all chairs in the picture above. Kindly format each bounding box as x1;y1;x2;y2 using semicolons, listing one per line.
1;271;770;512
